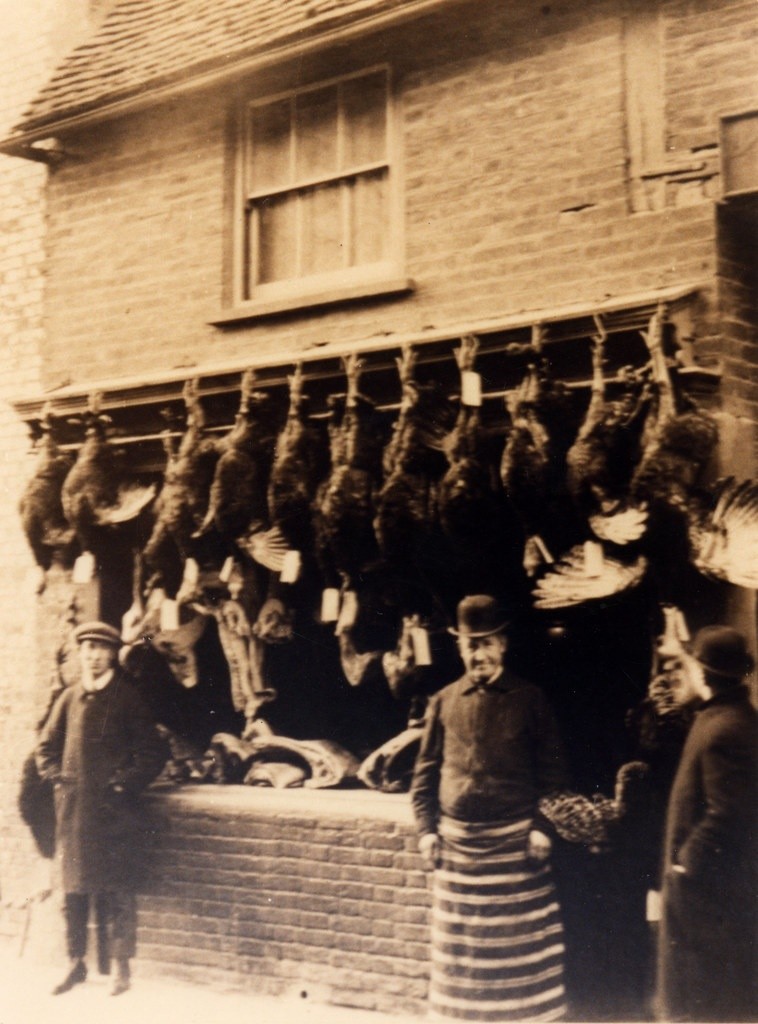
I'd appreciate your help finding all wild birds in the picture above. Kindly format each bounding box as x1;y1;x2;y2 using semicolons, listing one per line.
20;299;757;609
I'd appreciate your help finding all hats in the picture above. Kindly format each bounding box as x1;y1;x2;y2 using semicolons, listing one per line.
448;596;513;637
679;624;756;679
75;620;124;647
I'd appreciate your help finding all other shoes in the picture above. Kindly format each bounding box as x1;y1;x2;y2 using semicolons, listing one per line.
52;964;130;998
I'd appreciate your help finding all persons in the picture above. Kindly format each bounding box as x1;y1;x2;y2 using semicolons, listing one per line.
33;620;166;1004
408;599;573;1022
650;628;757;1023
